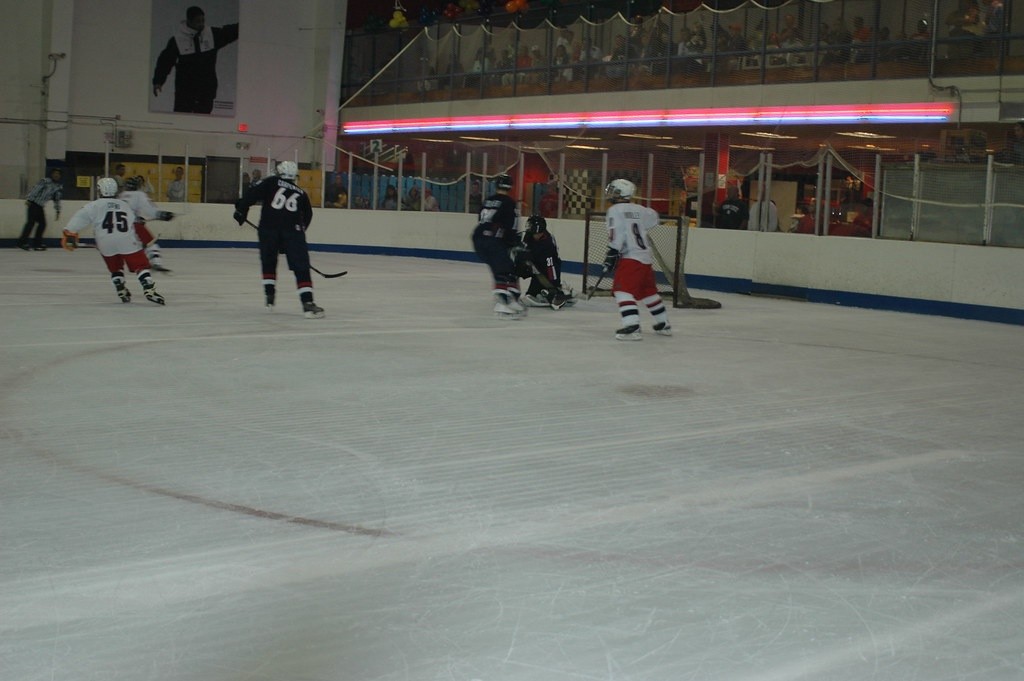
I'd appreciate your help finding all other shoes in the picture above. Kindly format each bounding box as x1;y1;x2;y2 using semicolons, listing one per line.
550;291;566;310
525;295;550;306
17;241;32;251
562;288;577;307
35;244;47;251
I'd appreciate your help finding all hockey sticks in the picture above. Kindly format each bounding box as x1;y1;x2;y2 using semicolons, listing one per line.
135;212;187;223
244;218;348;279
70;246;97;249
568;267;609;301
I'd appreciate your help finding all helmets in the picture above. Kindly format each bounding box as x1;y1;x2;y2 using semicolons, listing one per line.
525;216;547;233
277;161;298;179
124;178;137;191
97;177;118;197
606;179;637;199
495;174;513;189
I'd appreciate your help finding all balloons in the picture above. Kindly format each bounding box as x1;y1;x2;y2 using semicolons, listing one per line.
506;0;529;14
362;11;408;33
459;0;497;16
419;3;462;27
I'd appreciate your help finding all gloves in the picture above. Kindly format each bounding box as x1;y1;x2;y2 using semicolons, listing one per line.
233;203;249;226
61;229;79;252
602;252;619;274
159;211;173;221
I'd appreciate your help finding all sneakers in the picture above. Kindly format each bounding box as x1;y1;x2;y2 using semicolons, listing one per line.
616;324;642;340
653;320;672;335
507;286;527;317
154;266;175;275
492;289;517;319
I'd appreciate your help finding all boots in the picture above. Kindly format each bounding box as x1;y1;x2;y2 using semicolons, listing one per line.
298;287;325;318
137;269;165;305
112;276;131;301
263;280;275;313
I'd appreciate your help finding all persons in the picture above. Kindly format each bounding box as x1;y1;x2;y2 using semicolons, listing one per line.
243;169;262;205
380;184;398;210
325;174;348;209
468;183;481;214
407;187;421;211
167;167;185;202
600;178;674;339
61;178;166;305
113;164;127;191
516;215;579;309
120;175;176;276
716;185;750;231
747;191;778;232
414;16;739;93
425;189;441;211
539;181;558;218
470;175;530;321
153;6;240;115
17;170;62;250
233;161;326;319
135;175;156;193
741;0;1006;67
